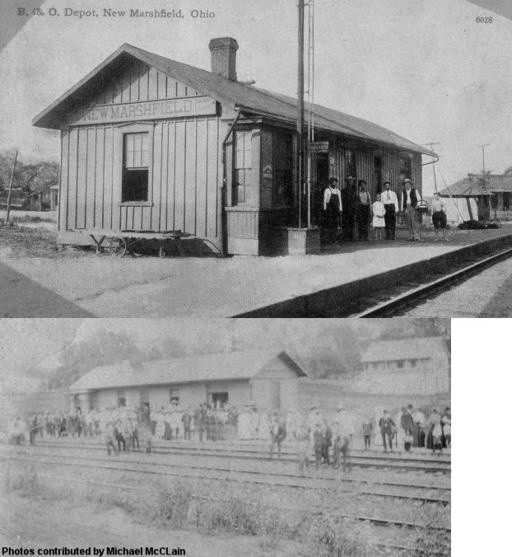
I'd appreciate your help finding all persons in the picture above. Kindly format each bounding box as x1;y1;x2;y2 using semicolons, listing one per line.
323;175;449;244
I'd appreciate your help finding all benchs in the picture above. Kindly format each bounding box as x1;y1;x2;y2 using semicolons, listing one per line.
75;229;194;257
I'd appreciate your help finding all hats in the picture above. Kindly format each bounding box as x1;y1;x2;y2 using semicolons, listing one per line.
328;176;412;185
